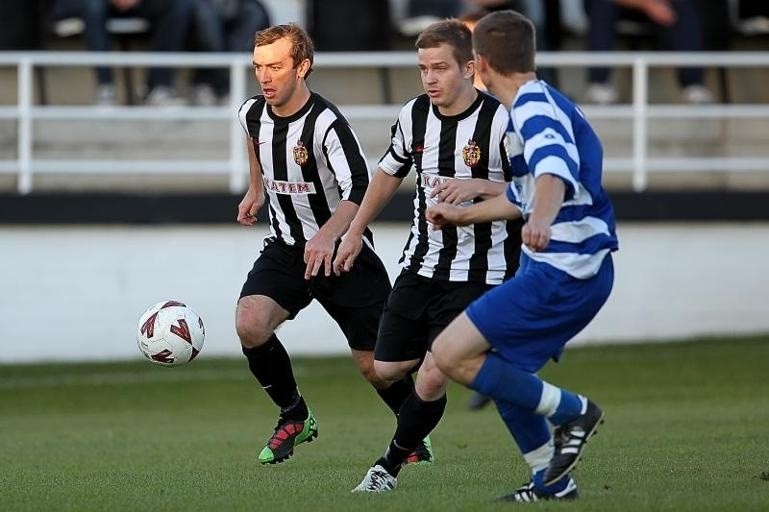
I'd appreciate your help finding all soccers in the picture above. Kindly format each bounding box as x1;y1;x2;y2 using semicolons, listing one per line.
136;301;205;367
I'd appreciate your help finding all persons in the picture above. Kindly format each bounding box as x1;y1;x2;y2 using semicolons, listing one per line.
331;16;524;496
442;11;501;415
235;21;435;467
423;9;619;504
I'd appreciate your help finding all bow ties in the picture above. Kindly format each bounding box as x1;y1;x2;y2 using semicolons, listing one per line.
93;83;220;109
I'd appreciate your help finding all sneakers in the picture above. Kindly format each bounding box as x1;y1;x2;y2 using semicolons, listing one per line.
350;455;402;494
402;429;437;467
256;407;319;466
585;81;713;104
538;393;604;488
494;473;576;503
